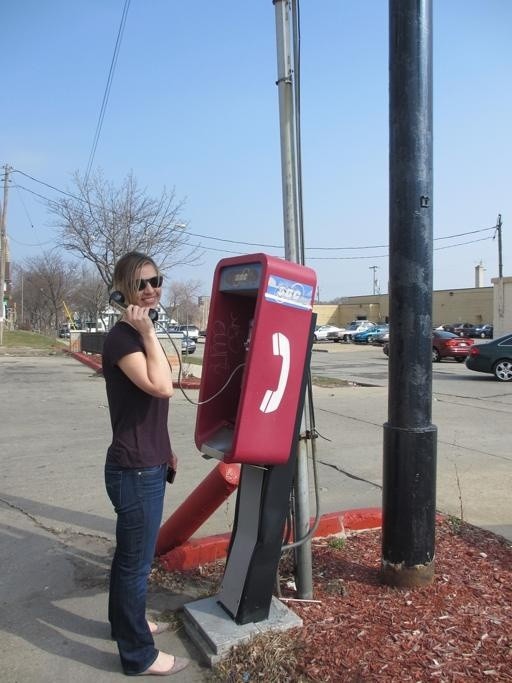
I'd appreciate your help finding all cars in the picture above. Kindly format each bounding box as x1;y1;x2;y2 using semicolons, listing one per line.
168;331;196;353
383;329;475;362
59;322;88;338
432;322;493;338
465;332;512;382
313;319;390;347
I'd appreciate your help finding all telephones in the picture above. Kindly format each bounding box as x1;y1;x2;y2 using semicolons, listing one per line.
109;291;158;325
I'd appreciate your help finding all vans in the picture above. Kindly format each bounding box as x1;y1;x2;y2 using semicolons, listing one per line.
168;325;200;343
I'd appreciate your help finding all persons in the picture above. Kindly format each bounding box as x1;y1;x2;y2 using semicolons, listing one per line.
105;251;191;676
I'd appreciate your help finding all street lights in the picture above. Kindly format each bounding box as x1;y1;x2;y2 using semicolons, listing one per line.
144;222;185;252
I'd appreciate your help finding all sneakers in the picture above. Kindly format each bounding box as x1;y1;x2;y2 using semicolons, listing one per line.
127;275;163;292
150;622;169;634
137;654;191;676
